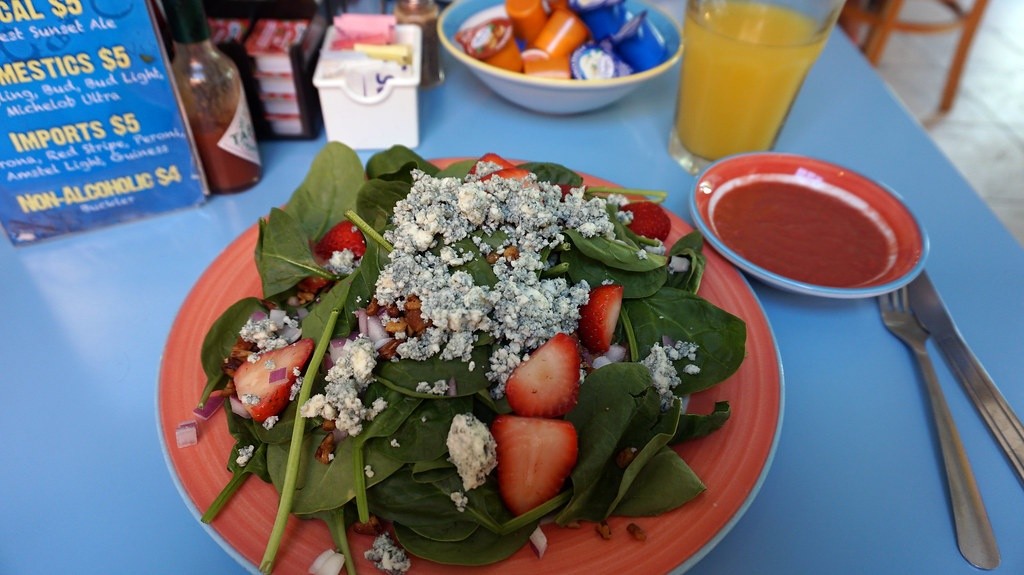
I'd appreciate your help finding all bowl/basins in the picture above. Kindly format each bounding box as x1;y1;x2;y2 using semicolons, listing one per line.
434;0;688;118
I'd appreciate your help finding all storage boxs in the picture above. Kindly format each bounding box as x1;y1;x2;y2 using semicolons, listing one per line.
313;22;423;154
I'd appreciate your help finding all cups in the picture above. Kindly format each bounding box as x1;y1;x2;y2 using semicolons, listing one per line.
670;0;845;172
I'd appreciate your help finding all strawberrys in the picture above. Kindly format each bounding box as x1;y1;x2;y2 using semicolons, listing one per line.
621;201;671;242
506;333;579;419
301;221;367;289
579;282;624;354
491;415;578;517
232;338;314;421
471;153;541;190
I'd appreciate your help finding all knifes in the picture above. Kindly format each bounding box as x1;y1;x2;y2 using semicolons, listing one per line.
908;270;1023;483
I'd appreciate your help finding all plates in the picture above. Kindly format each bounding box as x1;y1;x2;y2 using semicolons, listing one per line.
688;145;930;300
157;156;784;573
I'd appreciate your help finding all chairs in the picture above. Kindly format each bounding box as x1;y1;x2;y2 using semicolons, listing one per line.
837;1;987;111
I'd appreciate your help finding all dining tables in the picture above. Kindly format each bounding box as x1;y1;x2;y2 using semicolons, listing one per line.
0;0;1024;575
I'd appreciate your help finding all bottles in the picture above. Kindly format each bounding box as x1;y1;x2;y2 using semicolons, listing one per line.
165;3;266;193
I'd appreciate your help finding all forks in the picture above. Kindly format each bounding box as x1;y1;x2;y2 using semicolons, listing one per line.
875;281;997;570
